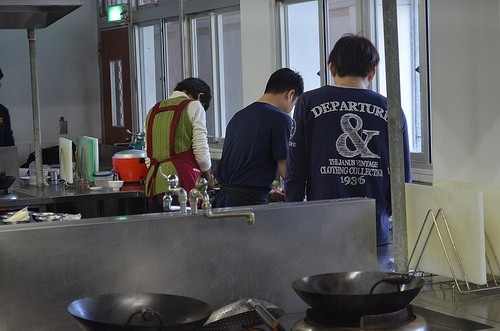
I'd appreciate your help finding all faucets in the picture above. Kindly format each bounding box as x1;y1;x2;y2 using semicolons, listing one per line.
188;177;255;225
162;173;191;214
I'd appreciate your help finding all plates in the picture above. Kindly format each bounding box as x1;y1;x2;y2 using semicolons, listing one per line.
89;187;102;191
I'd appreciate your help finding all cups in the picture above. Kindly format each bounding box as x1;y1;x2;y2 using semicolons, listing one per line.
76;177;90;194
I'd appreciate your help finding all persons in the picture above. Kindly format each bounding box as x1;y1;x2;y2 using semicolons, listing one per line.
213;67;305;209
0;70;16;148
284;31;414;274
143;77;217;217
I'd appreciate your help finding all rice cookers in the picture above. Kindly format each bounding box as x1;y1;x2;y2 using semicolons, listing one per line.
111;150;150;185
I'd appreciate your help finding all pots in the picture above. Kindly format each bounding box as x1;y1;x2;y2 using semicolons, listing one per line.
0;174;16;189
292;271;425;314
67;292;212;331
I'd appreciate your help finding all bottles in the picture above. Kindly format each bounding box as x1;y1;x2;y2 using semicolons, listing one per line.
28;161;37;185
43;165;60;181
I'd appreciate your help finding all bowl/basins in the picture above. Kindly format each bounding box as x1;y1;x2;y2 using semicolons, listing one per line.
108;181;124;191
1;209;67;224
92;171;115;188
18;167;28;177
